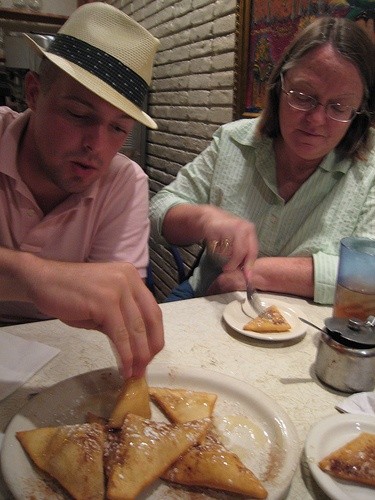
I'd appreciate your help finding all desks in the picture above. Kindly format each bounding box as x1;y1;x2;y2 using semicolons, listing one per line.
0;291;375;500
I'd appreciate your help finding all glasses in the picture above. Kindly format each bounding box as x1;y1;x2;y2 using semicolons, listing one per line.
279;71;363;123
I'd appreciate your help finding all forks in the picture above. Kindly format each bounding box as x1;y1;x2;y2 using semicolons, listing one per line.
238;263;268;320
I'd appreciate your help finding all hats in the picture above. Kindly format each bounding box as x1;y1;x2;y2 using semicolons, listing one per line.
22;3;160;131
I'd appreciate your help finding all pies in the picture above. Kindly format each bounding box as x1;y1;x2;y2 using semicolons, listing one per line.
15;373;267;500
318;433;375;485
243;305;291;332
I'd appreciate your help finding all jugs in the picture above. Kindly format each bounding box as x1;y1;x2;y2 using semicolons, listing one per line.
332;236;375;321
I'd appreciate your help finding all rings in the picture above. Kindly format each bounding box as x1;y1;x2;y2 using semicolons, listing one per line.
207;240;221;247
221;237;233;248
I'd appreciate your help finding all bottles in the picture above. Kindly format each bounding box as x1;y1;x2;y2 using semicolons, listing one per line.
315;317;375;394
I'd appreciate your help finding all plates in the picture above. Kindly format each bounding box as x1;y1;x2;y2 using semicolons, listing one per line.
223;297;307;340
304;413;374;500
1;363;299;500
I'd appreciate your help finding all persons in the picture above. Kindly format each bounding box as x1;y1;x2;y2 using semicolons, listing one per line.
0;2;165;380
147;17;375;304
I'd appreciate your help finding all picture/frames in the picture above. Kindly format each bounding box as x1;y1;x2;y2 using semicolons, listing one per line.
231;1;375;121
